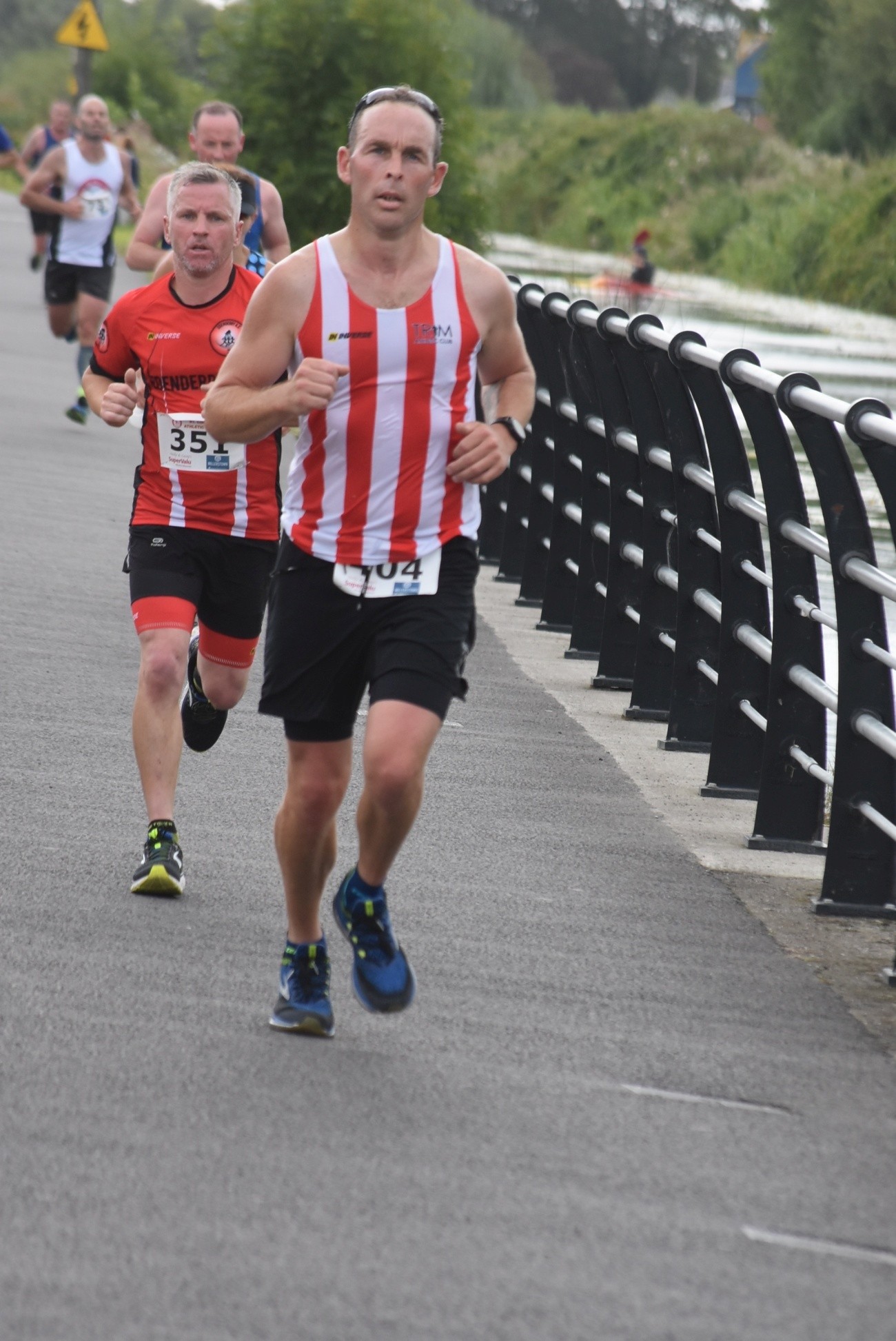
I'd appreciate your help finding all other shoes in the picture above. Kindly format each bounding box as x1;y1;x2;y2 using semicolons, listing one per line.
31;253;42;270
66;396;89;425
65;325;78;344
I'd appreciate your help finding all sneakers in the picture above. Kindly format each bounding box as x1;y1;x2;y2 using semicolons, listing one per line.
130;820;185;895
178;626;229;754
269;923;336;1039
333;868;417;1014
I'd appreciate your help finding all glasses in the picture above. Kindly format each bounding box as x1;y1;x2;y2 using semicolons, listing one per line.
351;86;440;128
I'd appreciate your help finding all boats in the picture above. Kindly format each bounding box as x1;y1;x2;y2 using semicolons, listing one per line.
572;272;679;298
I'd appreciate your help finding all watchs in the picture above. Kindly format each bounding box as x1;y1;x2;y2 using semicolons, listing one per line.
490;416;526;450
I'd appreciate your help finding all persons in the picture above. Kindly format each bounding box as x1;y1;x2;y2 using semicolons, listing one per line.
126;98;294;272
151;168;276;281
80;160;287;897
202;85;535;1037
0;95;141;427
